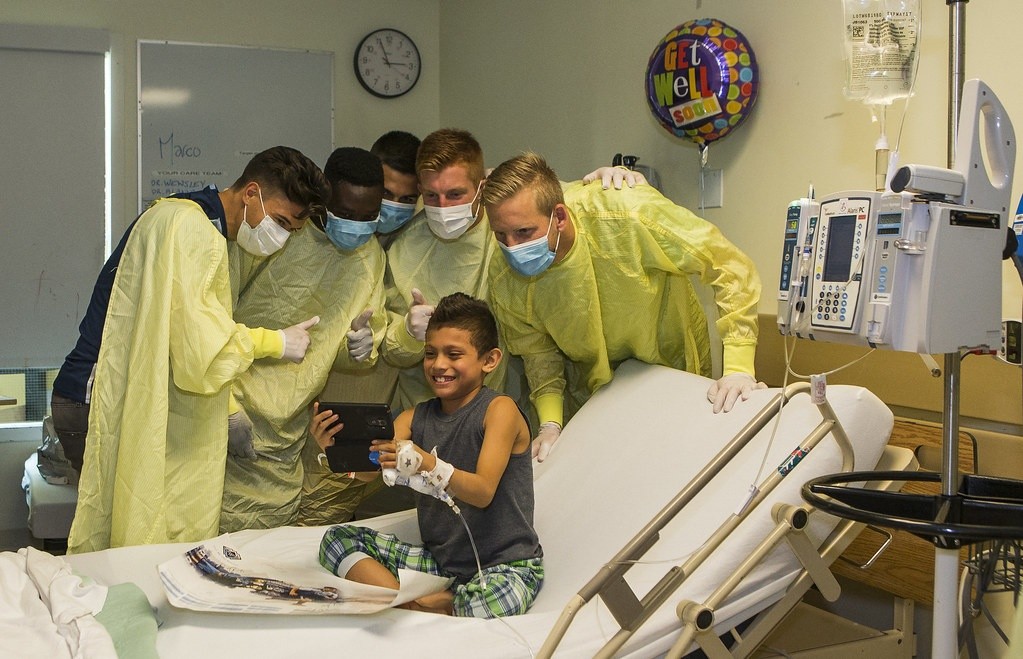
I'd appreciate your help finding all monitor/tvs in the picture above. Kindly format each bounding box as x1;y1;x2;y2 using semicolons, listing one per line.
824;215;856;282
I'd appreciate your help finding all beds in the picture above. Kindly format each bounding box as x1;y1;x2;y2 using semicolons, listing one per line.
0;358;921;659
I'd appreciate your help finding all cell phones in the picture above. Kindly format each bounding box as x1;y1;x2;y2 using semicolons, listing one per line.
318;402;395;474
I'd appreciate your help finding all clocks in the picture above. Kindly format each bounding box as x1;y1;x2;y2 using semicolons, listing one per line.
352;28;422;100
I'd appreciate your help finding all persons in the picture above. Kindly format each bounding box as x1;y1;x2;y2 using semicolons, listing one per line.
381;124;647;442
308;292;545;619
295;129;421;528
48;146;320;555
481;147;768;463
218;148;388;536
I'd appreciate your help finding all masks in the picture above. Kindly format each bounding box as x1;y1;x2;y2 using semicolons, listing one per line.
320;206;380;251
377;199;416;233
236;190;291;256
423;181;483;241
490;213;561;279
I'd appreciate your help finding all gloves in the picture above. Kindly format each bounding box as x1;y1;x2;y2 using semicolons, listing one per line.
283;314;320;364
581;166;644;189
346;311;374;363
228;411;258;461
405;288;435;342
708;373;768;413
530;422;561;462
